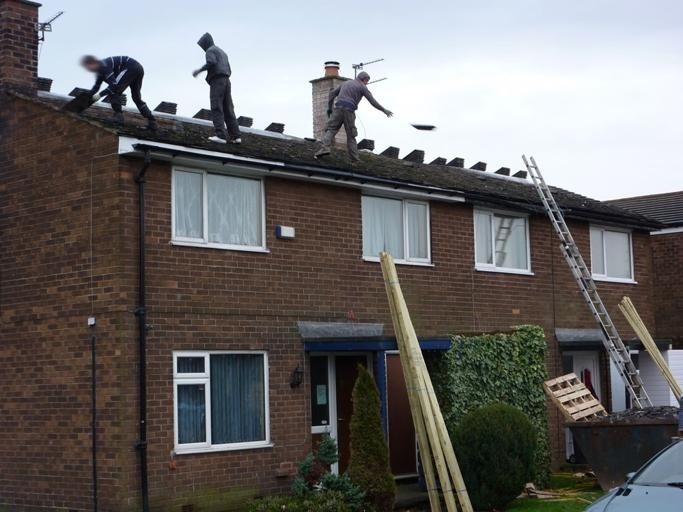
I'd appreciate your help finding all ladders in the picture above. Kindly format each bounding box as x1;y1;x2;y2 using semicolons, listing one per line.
522;154;653;409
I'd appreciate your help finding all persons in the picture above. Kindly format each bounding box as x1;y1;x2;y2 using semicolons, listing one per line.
314;71;395;165
81;53;159;130
192;32;242;145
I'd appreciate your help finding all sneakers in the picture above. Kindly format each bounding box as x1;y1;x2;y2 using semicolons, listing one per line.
103;111;124;125
208;136;243;143
142;119;160;131
314;145;331;157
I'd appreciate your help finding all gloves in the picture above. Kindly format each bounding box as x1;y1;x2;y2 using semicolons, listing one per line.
326;110;332;117
92;94;101;102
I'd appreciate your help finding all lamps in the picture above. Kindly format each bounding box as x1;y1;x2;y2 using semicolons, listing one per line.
291;363;304;388
